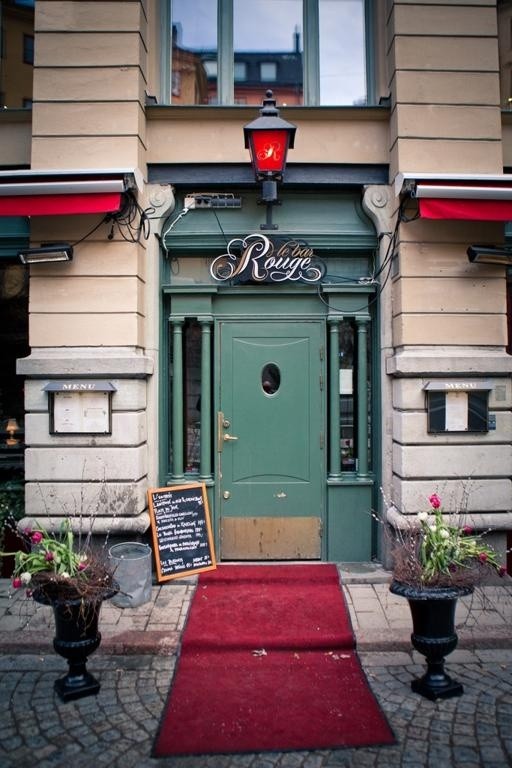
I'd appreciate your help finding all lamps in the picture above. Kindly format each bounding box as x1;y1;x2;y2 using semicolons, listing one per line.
243;90;298;230
465;245;511;267
18;246;73;265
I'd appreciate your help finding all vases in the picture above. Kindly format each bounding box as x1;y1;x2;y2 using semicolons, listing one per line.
32;581;120;703
389;581;476;702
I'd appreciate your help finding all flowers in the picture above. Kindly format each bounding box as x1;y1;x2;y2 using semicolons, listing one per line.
361;470;512;630
1;455;134;638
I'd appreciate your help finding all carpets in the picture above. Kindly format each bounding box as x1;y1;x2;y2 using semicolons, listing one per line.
150;562;399;758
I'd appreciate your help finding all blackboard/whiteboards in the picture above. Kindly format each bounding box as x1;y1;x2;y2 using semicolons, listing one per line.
148;483;217;582
